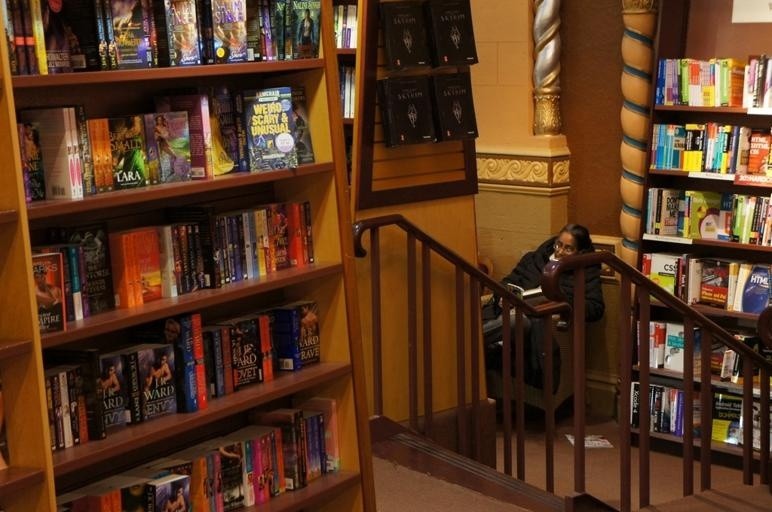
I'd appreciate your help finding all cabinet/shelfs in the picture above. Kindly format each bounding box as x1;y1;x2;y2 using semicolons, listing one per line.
1;1;377;512
629;53;771;474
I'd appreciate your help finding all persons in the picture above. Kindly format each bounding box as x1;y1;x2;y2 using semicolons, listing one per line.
482;221;606;428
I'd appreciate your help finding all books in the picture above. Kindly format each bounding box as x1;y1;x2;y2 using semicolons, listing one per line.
655;54;771;108
378;1;480;149
629;321;770;451
641;253;772;314
31;202;322;451
55;398;338;511
650;123;771;179
4;2;358;77
16;86;316;204
337;67;356;119
645;188;771;246
